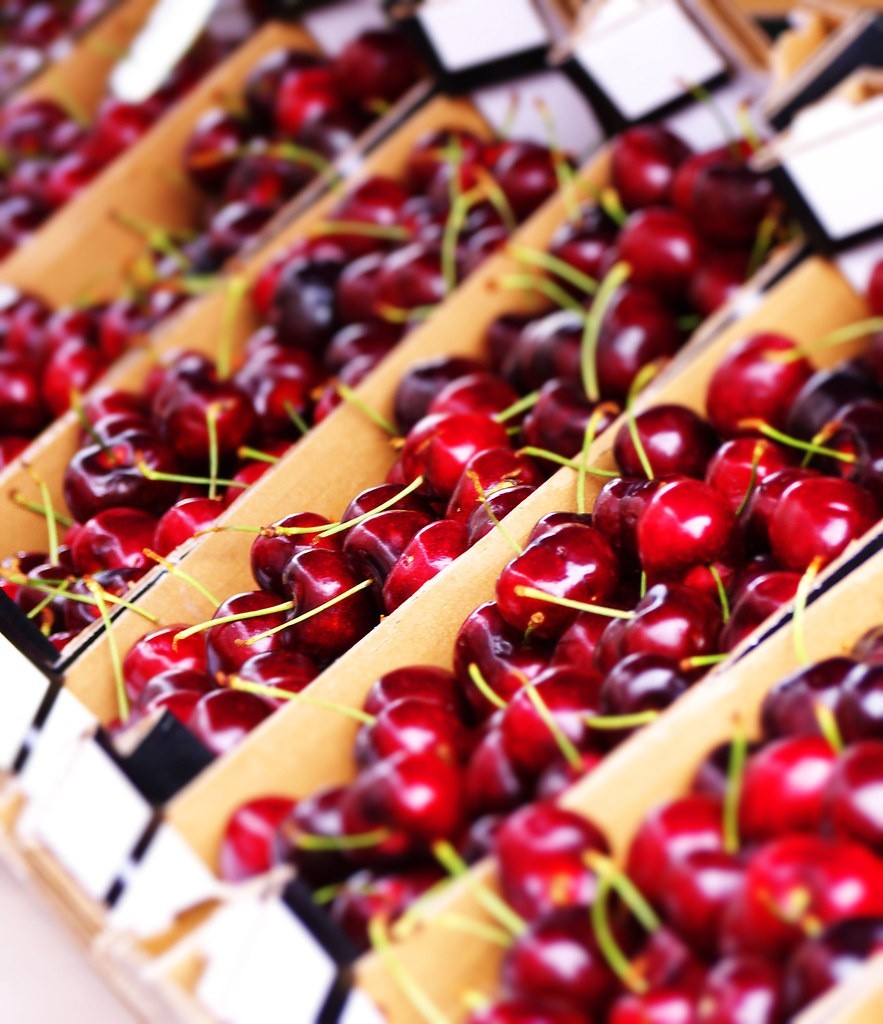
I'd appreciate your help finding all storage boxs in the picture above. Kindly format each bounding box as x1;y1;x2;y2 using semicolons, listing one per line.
0;0;883;1024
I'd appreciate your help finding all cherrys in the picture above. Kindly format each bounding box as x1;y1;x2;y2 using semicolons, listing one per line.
0;0;883;1024
